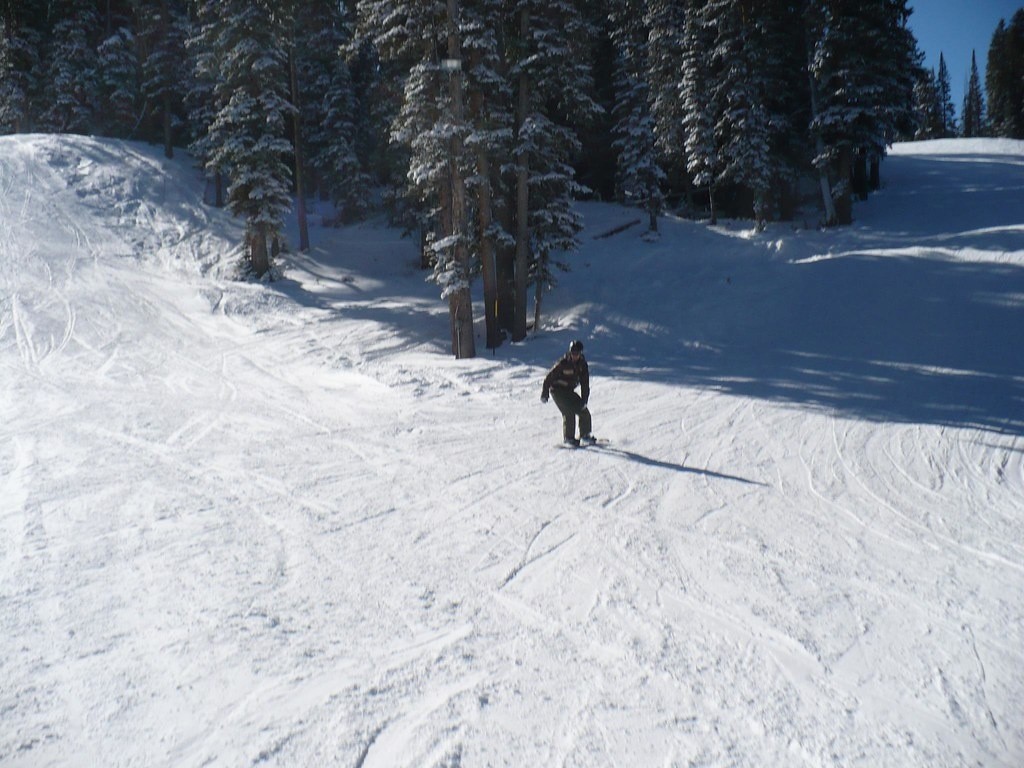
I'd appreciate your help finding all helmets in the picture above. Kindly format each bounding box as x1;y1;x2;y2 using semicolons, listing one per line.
569;340;583;352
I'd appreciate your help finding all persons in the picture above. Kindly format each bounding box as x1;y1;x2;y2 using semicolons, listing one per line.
540;340;597;447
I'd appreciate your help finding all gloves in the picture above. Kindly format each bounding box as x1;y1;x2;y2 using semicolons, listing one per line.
580;400;587;410
540;393;549;403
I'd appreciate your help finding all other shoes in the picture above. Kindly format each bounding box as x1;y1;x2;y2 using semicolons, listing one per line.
580;436;596;445
563;438;580;447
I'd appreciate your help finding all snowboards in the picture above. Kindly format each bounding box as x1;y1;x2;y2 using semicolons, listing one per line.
555;436;597;450
